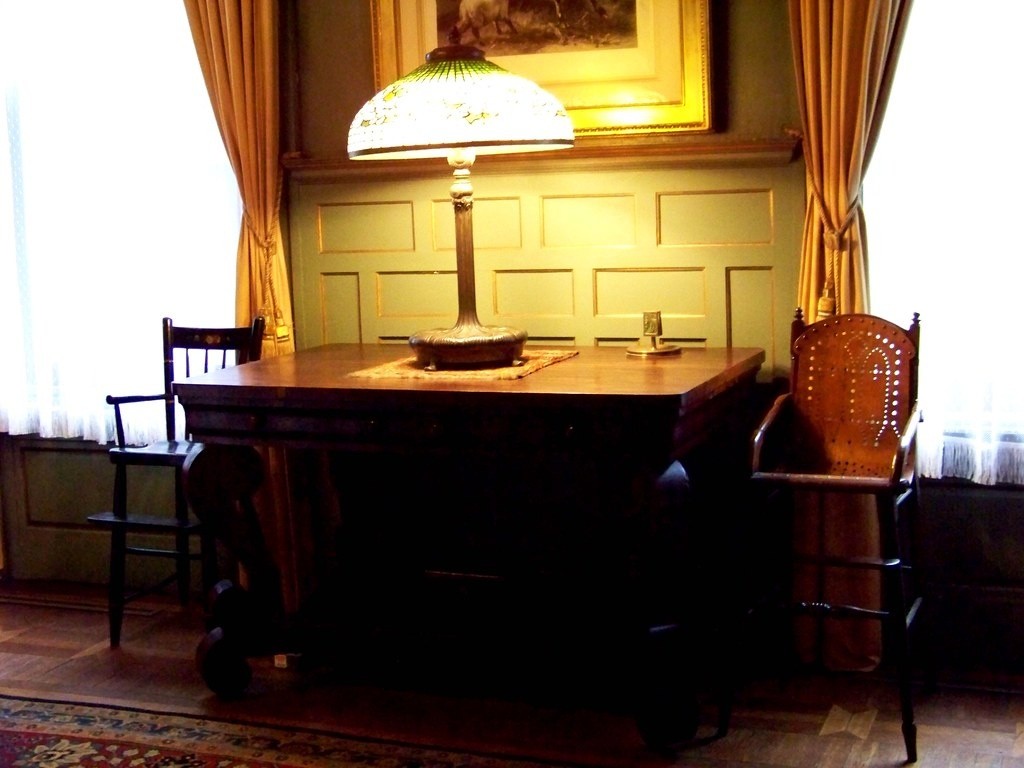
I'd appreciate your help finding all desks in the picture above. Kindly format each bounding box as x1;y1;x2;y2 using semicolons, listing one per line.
170;345;765;750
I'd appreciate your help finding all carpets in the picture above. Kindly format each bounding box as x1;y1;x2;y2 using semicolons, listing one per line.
0;694;587;768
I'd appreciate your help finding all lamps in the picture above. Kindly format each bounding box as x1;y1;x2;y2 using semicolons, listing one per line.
347;29;576;366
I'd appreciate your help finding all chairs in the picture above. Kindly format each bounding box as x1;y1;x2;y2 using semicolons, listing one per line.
88;317;266;643
716;307;920;762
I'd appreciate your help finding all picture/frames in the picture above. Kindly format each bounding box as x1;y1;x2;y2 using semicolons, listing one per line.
370;0;715;134
